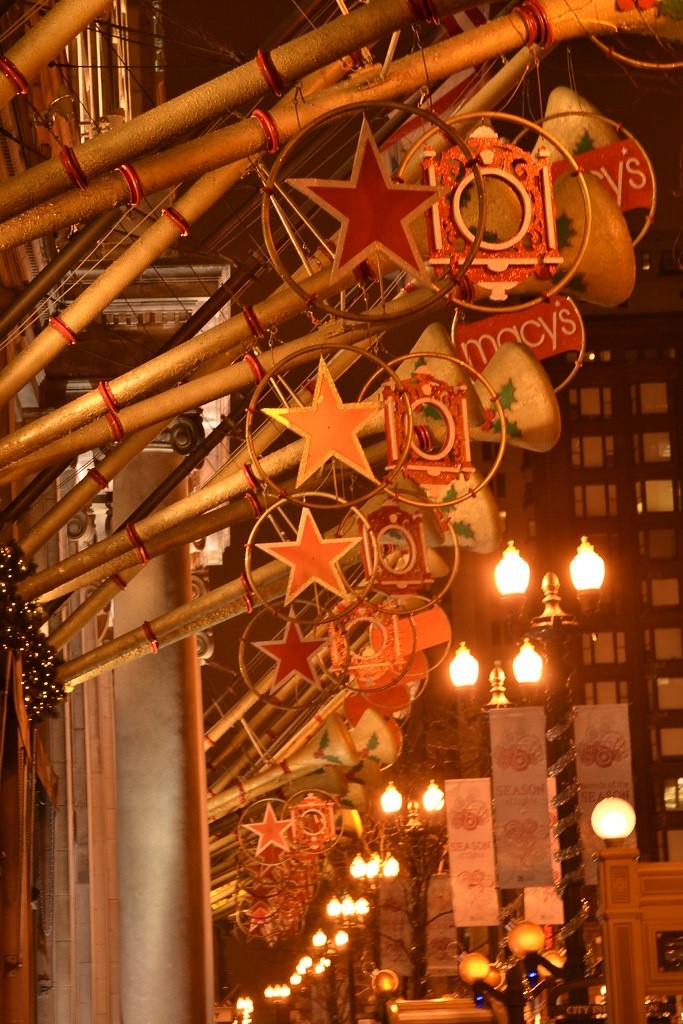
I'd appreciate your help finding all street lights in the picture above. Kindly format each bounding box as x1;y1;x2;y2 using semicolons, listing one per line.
350;851;400;1023
265;984;291;1023
312;929;350;1024
494;536;607;1023
326;892;369;1023
289;954;333;1024
379;780;446;999
450;634;546;1024
